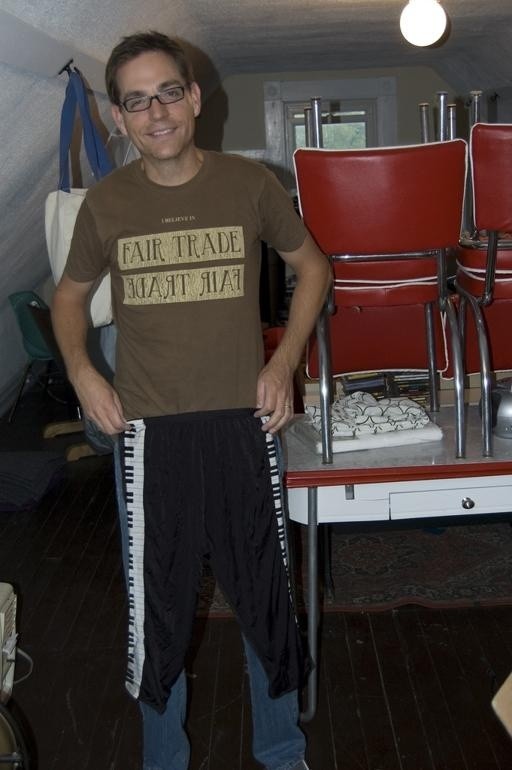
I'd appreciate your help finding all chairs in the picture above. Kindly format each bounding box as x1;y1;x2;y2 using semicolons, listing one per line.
0;290;70;425
443;123;511;457
291;138;468;460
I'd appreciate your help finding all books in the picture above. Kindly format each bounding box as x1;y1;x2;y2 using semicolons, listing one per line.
299;363;433;413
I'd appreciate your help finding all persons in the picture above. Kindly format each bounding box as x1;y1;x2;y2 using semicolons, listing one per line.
51;30;336;770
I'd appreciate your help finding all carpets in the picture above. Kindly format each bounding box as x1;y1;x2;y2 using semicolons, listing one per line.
191;514;512;618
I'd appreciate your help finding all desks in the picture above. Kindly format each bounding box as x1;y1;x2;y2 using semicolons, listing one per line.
280;401;512;724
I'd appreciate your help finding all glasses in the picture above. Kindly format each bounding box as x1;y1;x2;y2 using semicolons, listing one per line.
119;82;188;113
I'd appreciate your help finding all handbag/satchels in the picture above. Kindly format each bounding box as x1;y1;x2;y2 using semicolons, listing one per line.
44;71;114;330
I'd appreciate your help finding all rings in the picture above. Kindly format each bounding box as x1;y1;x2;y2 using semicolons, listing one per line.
284;404;291;409
